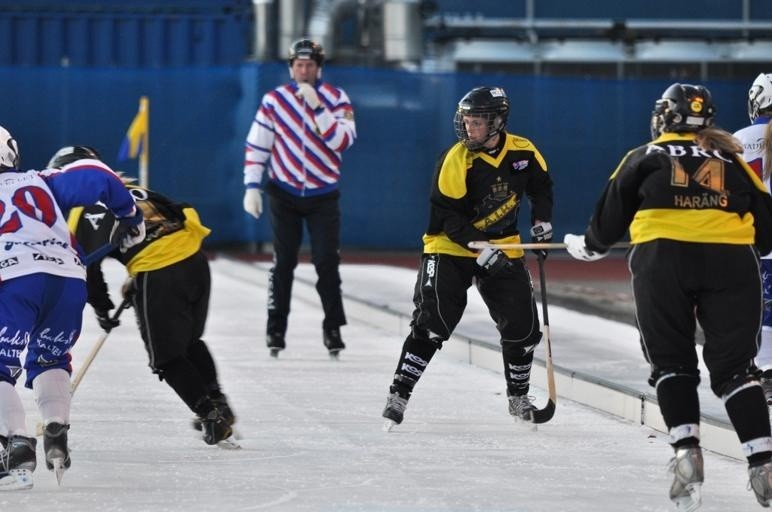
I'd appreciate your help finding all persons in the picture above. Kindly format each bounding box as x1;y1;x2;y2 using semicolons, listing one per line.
45;140;236;444
382;84;556;425
732;71;772;404
240;36;360;350
562;82;772;502
0;123;147;479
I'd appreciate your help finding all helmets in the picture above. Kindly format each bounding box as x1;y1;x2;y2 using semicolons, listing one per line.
745;72;772;124
47;145;103;171
286;38;325;65
453;86;510;152
0;127;22;174
648;82;717;141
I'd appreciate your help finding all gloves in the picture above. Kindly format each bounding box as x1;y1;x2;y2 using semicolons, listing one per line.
476;246;514;276
565;234;610;263
530;223;552;259
109;205;147;255
296;81;321;110
243;188;265;220
94;312;119;334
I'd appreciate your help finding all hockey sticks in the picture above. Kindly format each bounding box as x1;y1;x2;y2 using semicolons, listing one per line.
468;240;637;252
35;288;129;436
521;251;557;423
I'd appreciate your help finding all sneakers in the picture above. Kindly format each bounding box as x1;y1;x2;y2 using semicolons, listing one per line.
668;444;704;499
200;414;233;445
195;399;235;430
322;326;346;349
266;332;285;349
382;392;409;424
508;395;537;421
746;462;772;508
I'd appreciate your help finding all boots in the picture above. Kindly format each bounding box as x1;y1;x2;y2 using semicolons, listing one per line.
0;434;37;479
43;423;71;471
